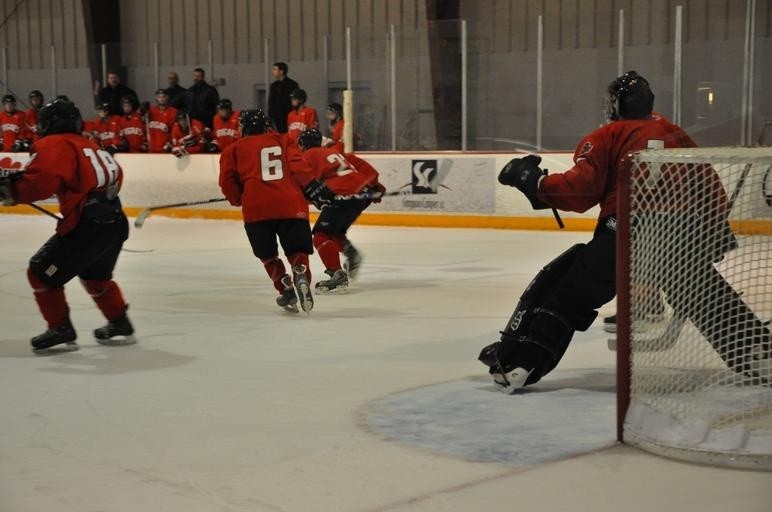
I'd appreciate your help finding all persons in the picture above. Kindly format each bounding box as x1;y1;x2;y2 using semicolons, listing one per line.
84;103;129;147
184;68;220;116
297;129;386;295
264;62;308;130
163;113;205;158
0;96;137;354
23;89;44;145
115;100;145;153
286;89;320;138
210;98;240;152
478;72;766;395
0;95;31;152
219;107;335;313
143;88;178;153
166;72;183;107
325;103;344;151
93;71;138;109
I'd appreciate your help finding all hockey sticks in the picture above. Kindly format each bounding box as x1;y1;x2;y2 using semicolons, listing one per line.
335;157;454;201
134;197;228;227
608;120;772;352
24;201;154;254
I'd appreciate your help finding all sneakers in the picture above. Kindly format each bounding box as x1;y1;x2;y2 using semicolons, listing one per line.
603;308;645;324
277;288;298;306
343;250;361;270
31;319;76;348
94;313;133;339
490;357;534;387
632;300;665;313
316;270;348;289
295;274;313;312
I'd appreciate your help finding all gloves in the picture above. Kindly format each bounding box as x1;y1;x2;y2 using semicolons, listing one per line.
703;226;726;261
373;184;385;203
23;139;31;151
499;155;541;191
162;141;173;152
174;147;184;154
140;142;149;150
181;135;198;146
0;141;3;151
10;139;22;151
305;178;336;209
117;141;128;151
107;144;116;152
206;142;218;152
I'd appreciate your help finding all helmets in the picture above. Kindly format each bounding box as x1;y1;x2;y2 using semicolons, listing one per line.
326;103;342;115
36;100;83;135
120;95;136;107
28;90;43;103
239;109;267;136
298;128;322;149
608;71;656;119
1;94;16;104
154;88;169;99
290;89;307;101
216;99;231;111
175;108;192;120
95;102;112;113
